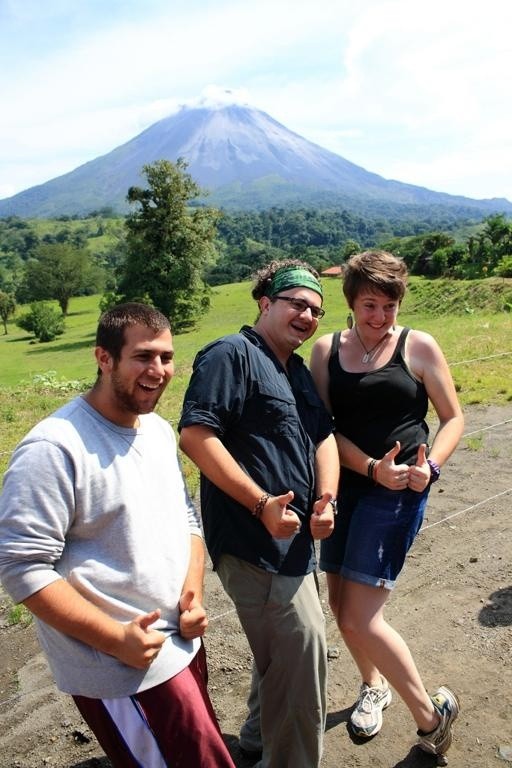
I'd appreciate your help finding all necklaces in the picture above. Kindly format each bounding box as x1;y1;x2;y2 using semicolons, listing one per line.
355;323;388;363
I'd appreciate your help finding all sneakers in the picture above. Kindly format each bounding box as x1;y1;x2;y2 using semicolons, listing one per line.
239;724;264;754
416;684;461;758
349;675;393;739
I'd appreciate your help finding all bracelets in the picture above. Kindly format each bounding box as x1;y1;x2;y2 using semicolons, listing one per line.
368;459;378;480
252;493;269;519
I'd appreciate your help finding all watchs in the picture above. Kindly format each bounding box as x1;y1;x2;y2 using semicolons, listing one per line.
427;459;440;483
315;496;338;515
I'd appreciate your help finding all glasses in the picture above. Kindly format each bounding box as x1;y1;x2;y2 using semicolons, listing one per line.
275;296;325;320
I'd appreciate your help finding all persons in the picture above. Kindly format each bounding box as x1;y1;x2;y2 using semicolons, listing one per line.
178;260;340;768
310;251;464;754
0;302;236;768
